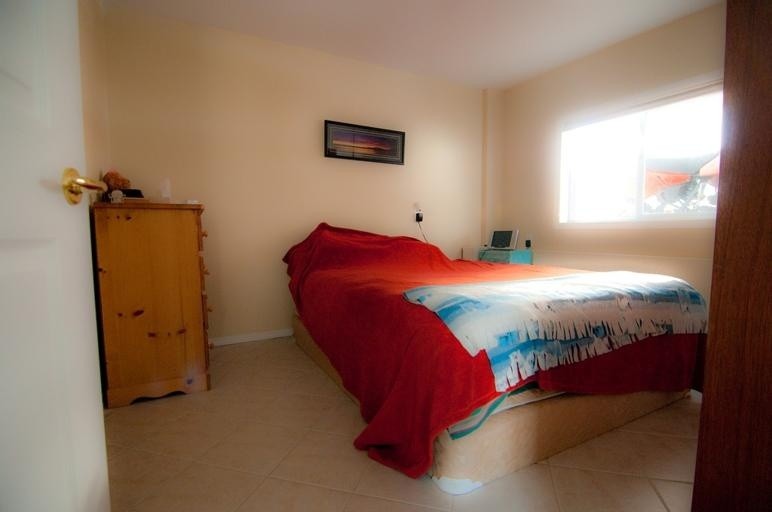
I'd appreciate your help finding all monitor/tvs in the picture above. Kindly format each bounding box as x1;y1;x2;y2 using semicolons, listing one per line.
487;227;519;249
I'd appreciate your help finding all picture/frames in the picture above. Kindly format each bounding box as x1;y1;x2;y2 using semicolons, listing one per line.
323;119;405;166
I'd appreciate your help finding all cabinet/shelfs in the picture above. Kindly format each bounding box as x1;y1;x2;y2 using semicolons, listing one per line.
90;202;215;408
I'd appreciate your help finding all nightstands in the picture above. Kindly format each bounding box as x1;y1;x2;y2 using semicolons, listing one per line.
477;249;533;265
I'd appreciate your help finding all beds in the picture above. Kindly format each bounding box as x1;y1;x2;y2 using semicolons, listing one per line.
286;223;706;494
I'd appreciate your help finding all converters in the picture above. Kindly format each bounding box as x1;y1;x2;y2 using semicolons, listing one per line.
526;240;531;247
416;213;423;222
